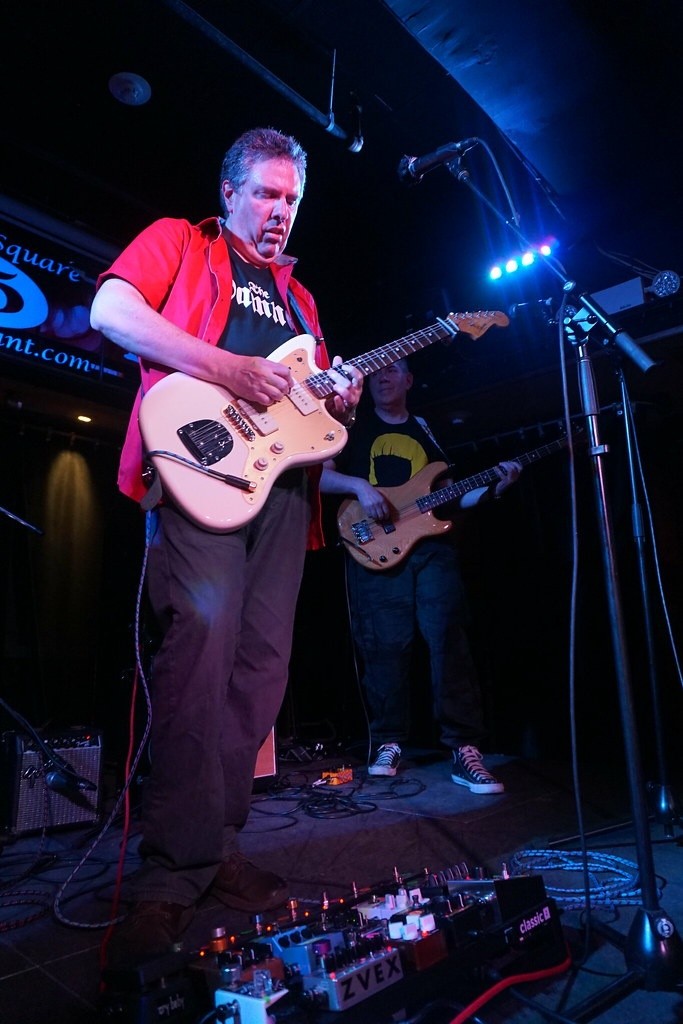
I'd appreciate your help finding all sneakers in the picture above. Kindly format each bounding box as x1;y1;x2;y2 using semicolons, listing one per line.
450;745;504;794
208;851;290;913
116;901;186;954
369;743;401;777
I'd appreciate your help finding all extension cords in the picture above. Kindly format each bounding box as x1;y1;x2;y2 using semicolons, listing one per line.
321;768;354;785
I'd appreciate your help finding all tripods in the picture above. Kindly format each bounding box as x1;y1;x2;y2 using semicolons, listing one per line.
436;136;682;1024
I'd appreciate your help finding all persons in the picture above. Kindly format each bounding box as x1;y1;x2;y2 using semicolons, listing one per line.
89;125;366;962
318;357;523;795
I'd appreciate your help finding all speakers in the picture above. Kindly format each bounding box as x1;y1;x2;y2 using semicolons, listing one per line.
0;732;102;835
252;724;280;793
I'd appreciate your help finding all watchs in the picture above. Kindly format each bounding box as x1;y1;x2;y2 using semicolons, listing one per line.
345;407;356;430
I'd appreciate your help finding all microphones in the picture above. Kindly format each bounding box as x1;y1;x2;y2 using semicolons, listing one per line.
45;772;96;791
508;296;554;319
397;137;478;185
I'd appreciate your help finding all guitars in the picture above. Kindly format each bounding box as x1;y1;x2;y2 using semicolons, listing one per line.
137;307;511;535
336;425;589;571
